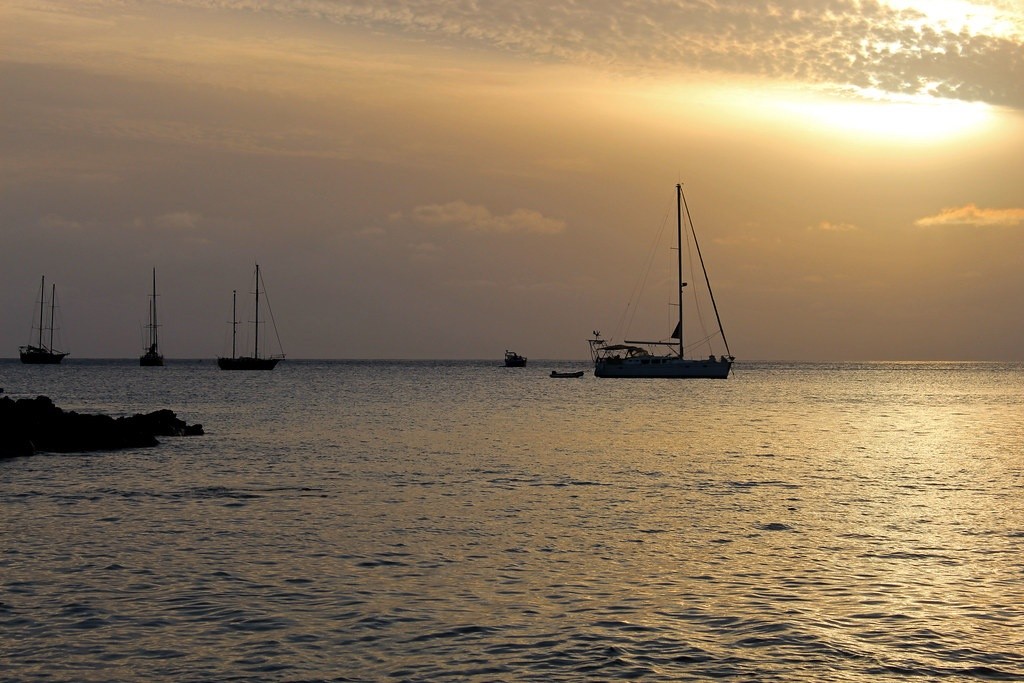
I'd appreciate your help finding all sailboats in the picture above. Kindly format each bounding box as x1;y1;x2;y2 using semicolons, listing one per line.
140;265;168;366
217;263;289;371
587;183;736;380
18;274;71;364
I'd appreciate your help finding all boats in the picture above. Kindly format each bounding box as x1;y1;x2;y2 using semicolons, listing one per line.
549;370;586;379
505;349;528;368
